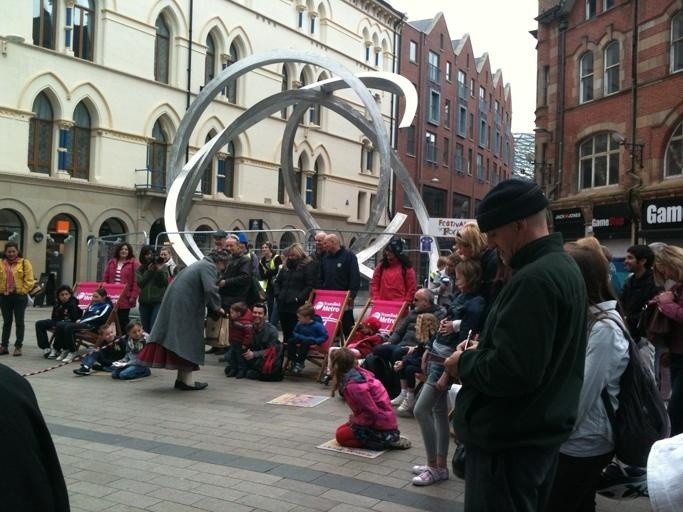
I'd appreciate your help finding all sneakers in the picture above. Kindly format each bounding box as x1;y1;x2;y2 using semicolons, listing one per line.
389;394;407;406
80;363;96;373
291;362;304;374
411;468;440;486
43;348;77;363
412;465;449;480
73;366;90;375
388;437;411;449
396;399;416;412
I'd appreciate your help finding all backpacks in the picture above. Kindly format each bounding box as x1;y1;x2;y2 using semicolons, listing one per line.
585;315;671;467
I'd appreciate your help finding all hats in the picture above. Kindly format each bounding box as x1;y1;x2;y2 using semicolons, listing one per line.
237;233;247;243
364;316;380;329
212;230;226;237
475;179;549;233
385;239;404;255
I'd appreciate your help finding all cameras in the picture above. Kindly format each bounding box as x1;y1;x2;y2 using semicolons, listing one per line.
150;255;164;263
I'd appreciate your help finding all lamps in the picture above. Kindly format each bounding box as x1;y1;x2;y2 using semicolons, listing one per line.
135;166;152;192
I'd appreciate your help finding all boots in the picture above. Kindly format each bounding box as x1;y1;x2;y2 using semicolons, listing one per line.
13;344;23;356
0;345;8;355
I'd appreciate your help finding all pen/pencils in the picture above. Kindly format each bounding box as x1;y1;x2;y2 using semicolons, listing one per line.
465;329;473;349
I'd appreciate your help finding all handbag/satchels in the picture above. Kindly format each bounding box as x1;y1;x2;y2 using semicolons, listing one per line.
205;311;231;349
635;304;670;334
248;276;267;304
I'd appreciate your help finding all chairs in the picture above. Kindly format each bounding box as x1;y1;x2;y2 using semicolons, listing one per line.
321;298;408;384
282;288;350;380
48;279;128;349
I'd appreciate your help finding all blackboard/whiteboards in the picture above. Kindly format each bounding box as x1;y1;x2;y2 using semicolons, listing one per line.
34;273;49;305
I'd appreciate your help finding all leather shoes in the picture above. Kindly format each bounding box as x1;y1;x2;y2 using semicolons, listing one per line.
174;379;200;388
235;370;244;378
227;369;236;377
179;381;208;390
320;375;334;383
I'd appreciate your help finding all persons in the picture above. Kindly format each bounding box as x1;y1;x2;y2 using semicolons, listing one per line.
133;243;233;392
535;236;632;512
0;242;34;356
328;347;412;448
205;231;360;382
370;224;681;492
34;233;181;383
442;178;589;511
